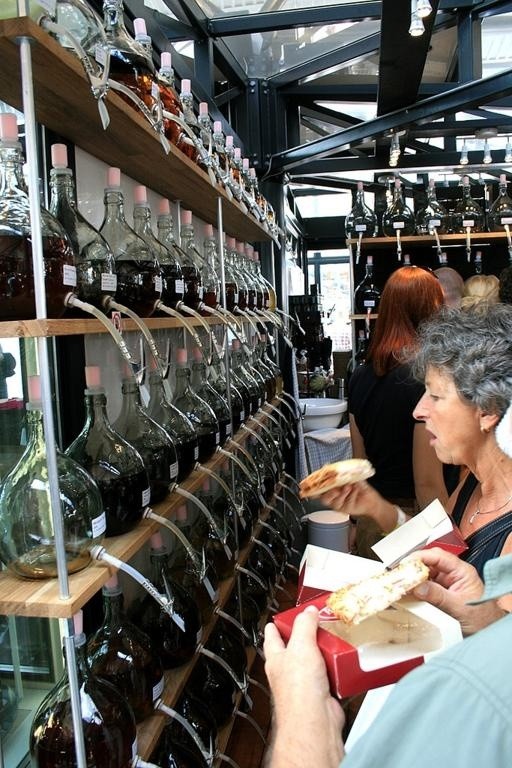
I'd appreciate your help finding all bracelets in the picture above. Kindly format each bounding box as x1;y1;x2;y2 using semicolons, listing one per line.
377;503;406;536
348;515;357;527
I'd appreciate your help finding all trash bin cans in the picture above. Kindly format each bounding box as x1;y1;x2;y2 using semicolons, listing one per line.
301;510;352;554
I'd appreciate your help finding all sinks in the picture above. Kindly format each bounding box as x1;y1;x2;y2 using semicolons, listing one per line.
300;397;347;432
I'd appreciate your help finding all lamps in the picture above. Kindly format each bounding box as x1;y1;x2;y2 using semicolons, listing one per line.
386;131;407;170
457;133;511;169
406;0;433;40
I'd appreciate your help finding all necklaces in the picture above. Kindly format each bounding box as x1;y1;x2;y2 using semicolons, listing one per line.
469;490;511;524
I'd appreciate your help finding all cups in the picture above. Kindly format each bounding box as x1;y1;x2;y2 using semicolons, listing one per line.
306;510;354;556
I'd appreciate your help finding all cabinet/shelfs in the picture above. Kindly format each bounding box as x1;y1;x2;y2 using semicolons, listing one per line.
1;11;314;768
341;176;510;366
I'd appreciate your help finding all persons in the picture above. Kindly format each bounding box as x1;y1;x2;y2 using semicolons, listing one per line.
264;403;512;768
463;275;500;307
498;263;512;307
297;297;512;586
347;266;446;551
433;266;465;308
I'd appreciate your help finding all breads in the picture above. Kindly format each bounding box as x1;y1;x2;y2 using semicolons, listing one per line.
296;458;377;497
326;557;430;625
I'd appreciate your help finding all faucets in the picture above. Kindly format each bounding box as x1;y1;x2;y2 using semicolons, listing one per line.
314;367;334;398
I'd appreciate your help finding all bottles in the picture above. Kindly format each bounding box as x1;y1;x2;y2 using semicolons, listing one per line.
292;347;299;366
0;330;276;585
337;376;345;398
344;175;512;236
348;251;511;373
16;0;212;132
0;120;278;318
300;349;310;368
27;485;296;766
